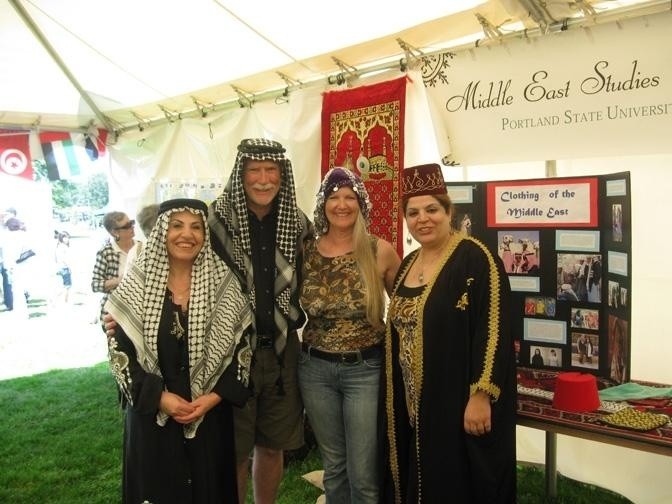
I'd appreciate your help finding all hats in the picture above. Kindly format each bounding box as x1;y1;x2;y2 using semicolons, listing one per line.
401;164;447;199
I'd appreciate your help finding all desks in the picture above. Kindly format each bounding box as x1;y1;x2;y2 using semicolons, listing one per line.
514;365;672;504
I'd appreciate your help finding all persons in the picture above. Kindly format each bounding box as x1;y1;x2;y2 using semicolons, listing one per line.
497;234;602;370
610;318;627;384
377;163;518;504
102;138;315;503
0;204;162;333
105;197;258;504
299;166;403;503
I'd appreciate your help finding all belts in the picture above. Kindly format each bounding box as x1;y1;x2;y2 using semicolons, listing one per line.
303;337;384;366
256;335;274;349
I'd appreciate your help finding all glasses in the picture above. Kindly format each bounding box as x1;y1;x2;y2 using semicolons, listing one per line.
115;220;135;229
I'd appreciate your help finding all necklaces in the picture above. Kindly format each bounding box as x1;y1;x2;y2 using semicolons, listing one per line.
166;279;191;300
418;237;451;283
